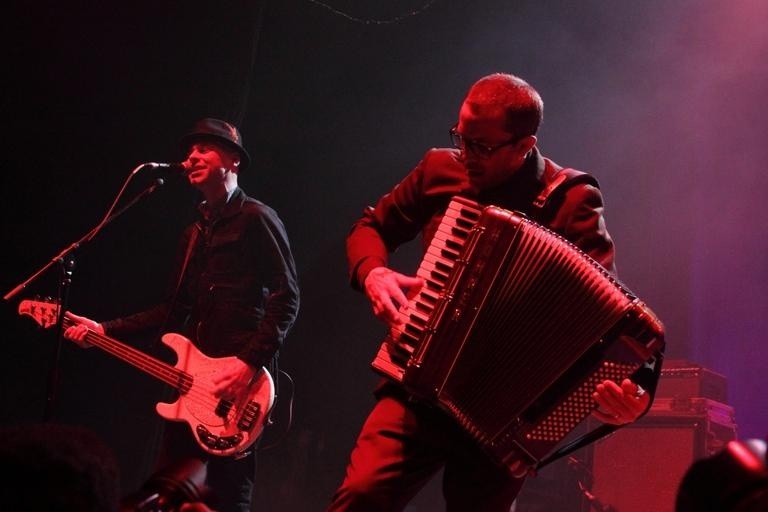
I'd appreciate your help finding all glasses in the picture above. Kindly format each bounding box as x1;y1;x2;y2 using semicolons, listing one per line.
449;123;518;158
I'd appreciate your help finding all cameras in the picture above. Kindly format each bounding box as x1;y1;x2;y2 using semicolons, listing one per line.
122;454;208;512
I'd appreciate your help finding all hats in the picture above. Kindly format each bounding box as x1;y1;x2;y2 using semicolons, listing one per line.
176;116;250;170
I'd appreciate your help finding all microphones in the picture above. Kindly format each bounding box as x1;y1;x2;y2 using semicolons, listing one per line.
149;178;164;193
147;160;186;174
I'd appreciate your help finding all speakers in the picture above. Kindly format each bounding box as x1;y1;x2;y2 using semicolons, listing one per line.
583;414;737;512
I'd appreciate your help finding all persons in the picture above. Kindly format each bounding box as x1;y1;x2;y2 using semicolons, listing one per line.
57;115;303;511
324;71;667;510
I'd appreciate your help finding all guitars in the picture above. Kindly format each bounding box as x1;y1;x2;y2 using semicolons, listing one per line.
18;294;275;456
